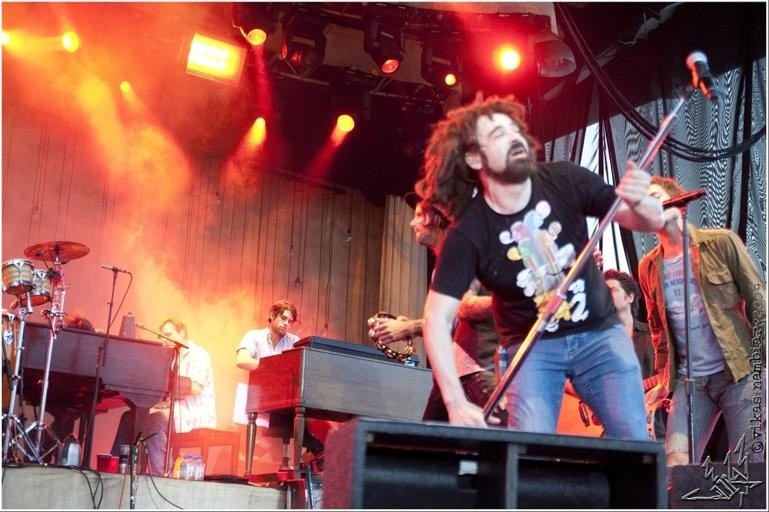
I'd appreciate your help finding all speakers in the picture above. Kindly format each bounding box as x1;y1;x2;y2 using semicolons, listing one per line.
322;416;667;509
671;463;766;509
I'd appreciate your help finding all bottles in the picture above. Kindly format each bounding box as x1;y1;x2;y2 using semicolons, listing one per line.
119;310;136;338
176;453;206;481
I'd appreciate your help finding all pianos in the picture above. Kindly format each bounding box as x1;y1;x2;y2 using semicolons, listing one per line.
246;336;435;480
10;321;175;475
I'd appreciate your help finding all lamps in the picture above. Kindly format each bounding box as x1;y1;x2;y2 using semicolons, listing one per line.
272;12;463;91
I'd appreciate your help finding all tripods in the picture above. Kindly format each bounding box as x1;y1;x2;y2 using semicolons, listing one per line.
2;284;64;465
2;307;46;467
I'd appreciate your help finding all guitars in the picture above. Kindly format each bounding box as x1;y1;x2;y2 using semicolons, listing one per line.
555;371;659;438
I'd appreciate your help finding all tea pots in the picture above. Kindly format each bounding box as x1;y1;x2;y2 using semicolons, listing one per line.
59;433;83;467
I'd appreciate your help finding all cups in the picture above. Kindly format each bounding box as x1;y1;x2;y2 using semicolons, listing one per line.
97;444;139;474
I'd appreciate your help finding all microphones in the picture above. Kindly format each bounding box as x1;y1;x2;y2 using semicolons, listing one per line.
685;49;719;100
662;188;707;210
101;263;127;273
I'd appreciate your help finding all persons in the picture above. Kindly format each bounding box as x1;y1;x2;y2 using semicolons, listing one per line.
419;89;664;443
236;300;324;456
562;269;669;445
367;192;480;422
458;242;604;420
637;176;766;465
111;319;217;477
47;319;96;445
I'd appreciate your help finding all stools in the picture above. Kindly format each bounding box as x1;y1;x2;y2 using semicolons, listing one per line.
165;425;243;482
246;422;316;487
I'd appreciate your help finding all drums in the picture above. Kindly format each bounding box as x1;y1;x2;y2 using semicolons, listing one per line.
19;270;51;307
2;308;24;410
2;259;33;294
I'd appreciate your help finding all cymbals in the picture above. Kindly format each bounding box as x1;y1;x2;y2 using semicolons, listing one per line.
24;241;90;264
